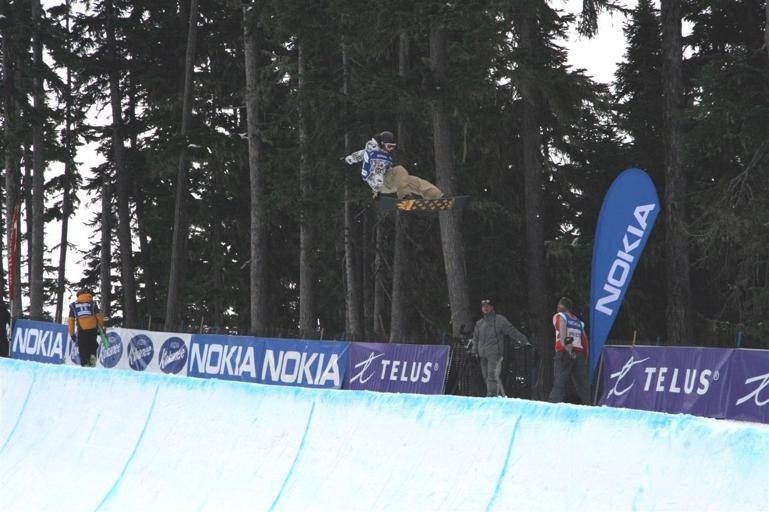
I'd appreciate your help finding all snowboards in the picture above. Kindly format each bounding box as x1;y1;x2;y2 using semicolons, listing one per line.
396;195;471;210
96;322;111;349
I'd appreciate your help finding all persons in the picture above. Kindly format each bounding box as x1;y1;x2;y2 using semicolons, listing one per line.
68;289;104;368
548;296;592;405
344;131;451;201
471;297;530;397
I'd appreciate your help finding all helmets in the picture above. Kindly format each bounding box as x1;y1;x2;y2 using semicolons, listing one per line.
378;130;396;145
